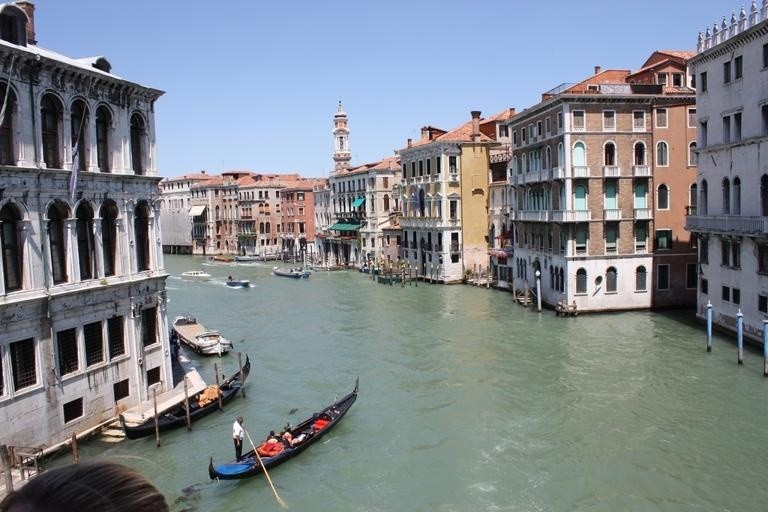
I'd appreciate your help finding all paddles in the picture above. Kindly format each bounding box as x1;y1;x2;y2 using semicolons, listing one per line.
245;430;288;509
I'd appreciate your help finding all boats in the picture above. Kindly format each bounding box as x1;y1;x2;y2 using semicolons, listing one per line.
359;265;382;274
178;270;213;280
224;279;251;286
208;378;361;479
174;315;231;356
272;265;305;279
208;252;286;262
116;354;250;439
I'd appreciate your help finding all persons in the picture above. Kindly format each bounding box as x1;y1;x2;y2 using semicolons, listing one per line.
282;425;306;446
232;415;245;462
266;430;281;442
0;461;170;512
